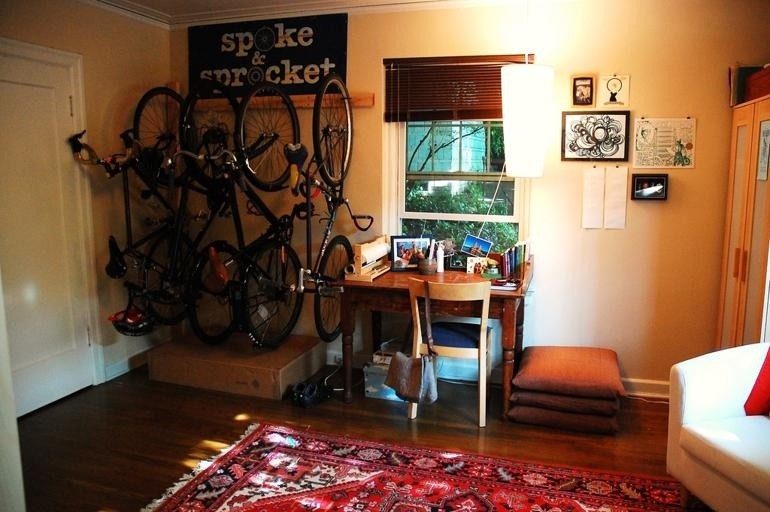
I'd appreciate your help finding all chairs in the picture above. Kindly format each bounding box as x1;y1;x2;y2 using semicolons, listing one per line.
407;277;493;427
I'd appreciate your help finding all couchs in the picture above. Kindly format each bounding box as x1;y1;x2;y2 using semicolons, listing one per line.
666;343;770;512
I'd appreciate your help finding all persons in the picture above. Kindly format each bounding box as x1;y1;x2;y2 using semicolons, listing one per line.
399;244;417;262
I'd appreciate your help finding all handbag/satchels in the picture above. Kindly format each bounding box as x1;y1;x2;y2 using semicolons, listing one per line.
383;351;439;404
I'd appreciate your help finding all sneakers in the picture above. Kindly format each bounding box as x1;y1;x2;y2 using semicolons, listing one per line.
301;382;321;408
291;382;309;405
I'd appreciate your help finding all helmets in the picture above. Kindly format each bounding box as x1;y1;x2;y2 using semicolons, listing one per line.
112;307;157;337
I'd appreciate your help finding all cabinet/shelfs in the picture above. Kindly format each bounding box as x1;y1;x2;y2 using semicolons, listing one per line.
715;95;770;350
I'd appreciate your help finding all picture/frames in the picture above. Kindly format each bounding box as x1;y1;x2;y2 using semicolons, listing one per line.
569;72;597;108
391;236;433;272
561;111;630;162
631;174;667;200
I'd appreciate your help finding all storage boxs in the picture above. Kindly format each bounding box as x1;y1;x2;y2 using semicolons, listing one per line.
363;361;409;403
744;66;770;102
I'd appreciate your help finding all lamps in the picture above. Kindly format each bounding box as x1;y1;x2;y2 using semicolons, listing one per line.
501;50;554;177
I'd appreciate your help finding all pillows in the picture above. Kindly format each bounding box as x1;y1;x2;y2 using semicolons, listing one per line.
506;405;620;435
512;346;627;399
743;346;770;416
509;390;620;418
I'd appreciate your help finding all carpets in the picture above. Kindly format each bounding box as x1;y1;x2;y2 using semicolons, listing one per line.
140;422;690;512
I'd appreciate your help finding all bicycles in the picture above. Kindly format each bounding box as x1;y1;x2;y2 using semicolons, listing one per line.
108;80;246;346
168;81;306;349
68;85;201;339
283;75;374;342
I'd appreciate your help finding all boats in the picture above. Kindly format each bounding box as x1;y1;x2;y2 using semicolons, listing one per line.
470;242;486;257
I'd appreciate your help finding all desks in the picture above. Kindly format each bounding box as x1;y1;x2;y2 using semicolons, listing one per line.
329;269;533;422
